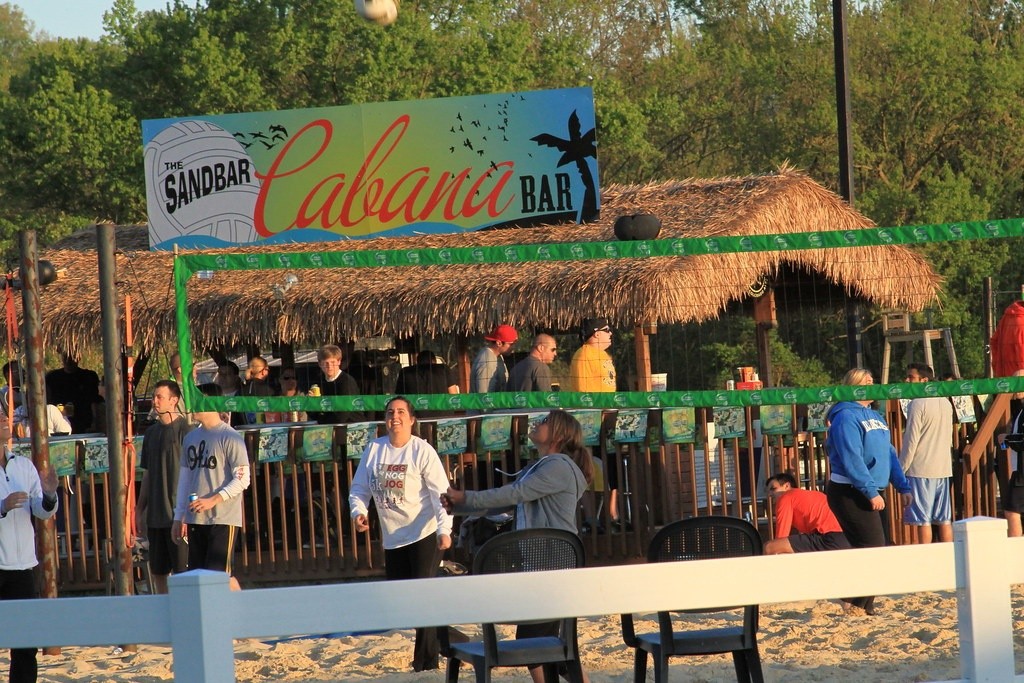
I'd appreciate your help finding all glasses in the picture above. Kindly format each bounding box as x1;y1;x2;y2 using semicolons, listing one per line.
596;327;609;334
13;369;26;374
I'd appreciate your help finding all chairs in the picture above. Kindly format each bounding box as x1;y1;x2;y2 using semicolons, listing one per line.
619;514;766;683
444;528;588;683
879;311;962;384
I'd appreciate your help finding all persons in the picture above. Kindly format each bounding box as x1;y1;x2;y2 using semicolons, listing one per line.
137;379;188;597
440;409;592;683
996;370;1024;538
0;316;622;523
762;473;853;555
990;271;1024;420
0;401;59;683
897;363;952;547
171;382;251;595
348;396;471;672
825;367;908;618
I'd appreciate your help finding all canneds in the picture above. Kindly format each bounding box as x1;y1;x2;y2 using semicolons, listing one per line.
745;511;751;521
726;379;735;391
189;495;199;512
291;411;298;422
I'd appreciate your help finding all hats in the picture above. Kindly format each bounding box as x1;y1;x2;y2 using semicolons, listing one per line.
484;325;518;343
580;315;608;344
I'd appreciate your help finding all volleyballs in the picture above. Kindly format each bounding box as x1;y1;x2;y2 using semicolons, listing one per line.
356;0;400;23
